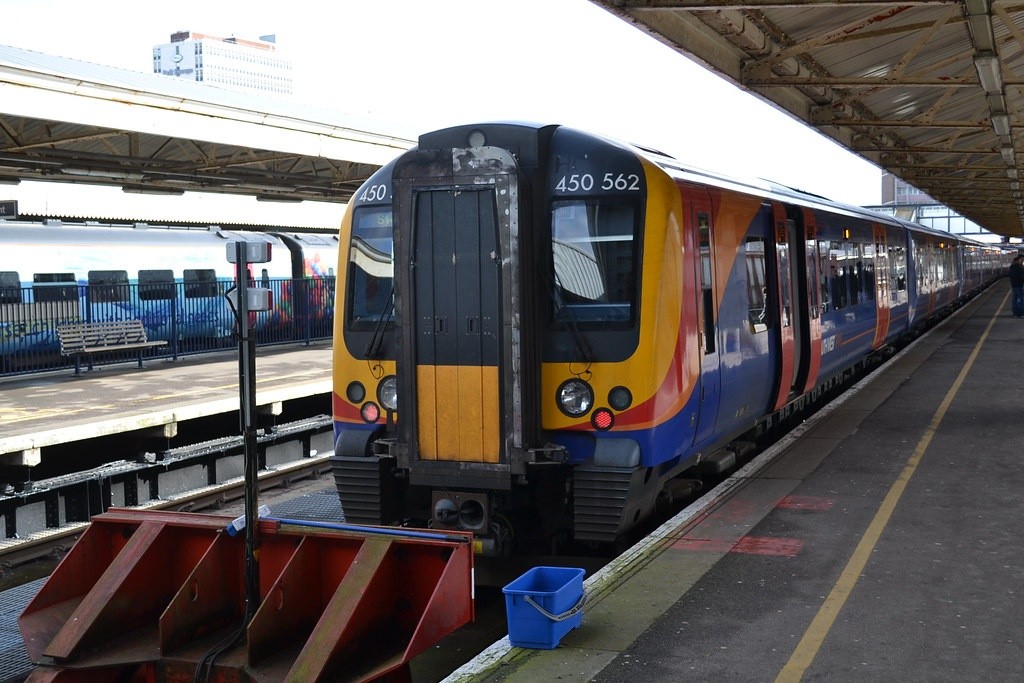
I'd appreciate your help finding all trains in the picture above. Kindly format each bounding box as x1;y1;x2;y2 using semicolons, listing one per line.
1;212;393;375
328;119;1024;559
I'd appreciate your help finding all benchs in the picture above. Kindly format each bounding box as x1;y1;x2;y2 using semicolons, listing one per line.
56;320;168;378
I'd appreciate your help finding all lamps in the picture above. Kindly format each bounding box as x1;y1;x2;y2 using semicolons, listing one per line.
272;402;283;415
164;423;178;437
22;448;42;467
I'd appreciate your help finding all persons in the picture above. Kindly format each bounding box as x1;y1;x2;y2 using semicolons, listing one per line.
1009;253;1024;318
